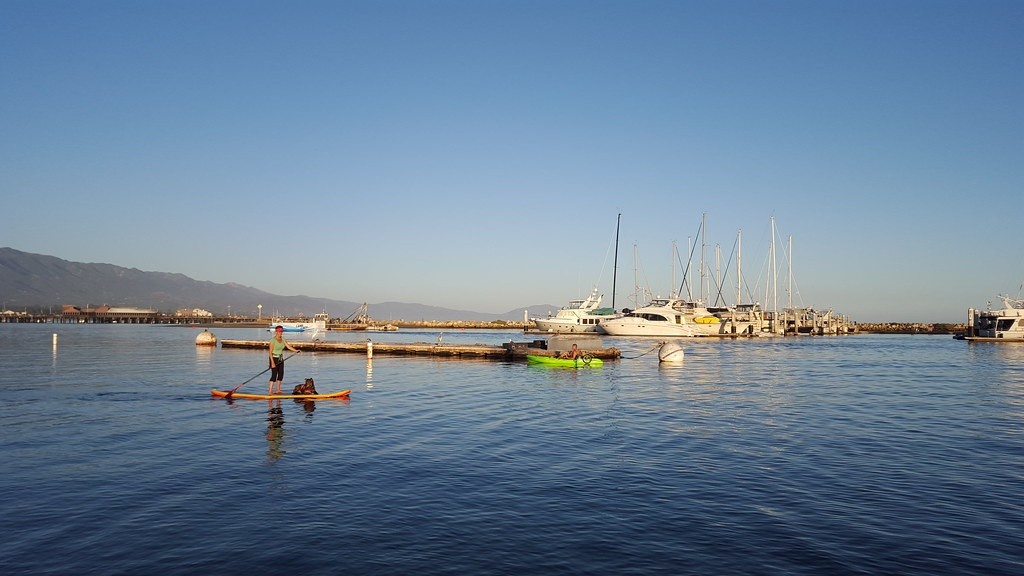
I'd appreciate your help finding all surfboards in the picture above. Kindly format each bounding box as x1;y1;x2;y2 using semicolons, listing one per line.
210;388;351;400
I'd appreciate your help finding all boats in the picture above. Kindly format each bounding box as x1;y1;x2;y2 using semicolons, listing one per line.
268;301;400;333
964;285;1024;343
505;340;621;360
527;355;603;367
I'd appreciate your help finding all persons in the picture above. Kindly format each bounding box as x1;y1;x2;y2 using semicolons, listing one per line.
268;326;302;396
557;344;579;360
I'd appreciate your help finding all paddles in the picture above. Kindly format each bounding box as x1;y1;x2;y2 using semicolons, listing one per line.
224;352;297;399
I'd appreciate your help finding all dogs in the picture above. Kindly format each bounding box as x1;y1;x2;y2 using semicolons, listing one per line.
292;378;318;395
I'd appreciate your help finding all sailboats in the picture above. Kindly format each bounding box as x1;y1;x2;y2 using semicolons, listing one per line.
523;207;795;337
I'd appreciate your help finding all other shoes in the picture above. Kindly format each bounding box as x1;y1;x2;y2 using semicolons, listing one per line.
269;391;277;395
275;391;287;395
